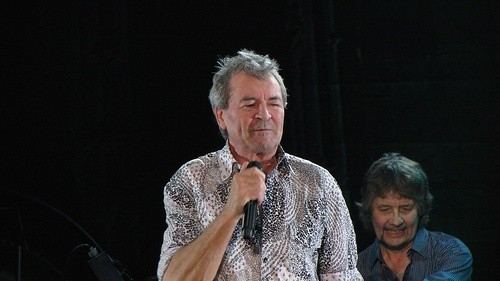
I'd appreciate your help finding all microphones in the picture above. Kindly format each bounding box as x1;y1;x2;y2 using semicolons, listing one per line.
87;246;124;281
243;161;262;240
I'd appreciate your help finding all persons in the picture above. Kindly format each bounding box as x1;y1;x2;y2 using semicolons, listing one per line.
155;50;367;280
356;152;473;281
